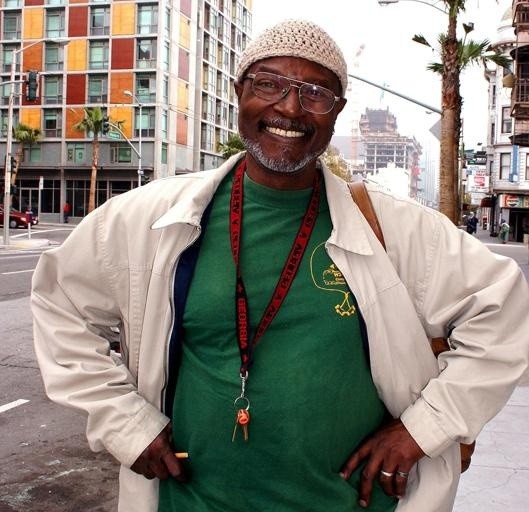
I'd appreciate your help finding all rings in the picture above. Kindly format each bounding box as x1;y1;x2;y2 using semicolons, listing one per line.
381;469;395;478
396;469;410;478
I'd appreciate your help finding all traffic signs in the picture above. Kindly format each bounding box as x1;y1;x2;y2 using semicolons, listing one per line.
108;131;120;140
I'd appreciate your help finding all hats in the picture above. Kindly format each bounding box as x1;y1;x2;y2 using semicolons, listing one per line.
230;20;349;83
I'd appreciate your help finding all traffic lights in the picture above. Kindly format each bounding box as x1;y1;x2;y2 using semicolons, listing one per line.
28;73;37;99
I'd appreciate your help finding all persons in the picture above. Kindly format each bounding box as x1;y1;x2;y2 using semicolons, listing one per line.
24;205;37;241
498;218;510;243
30;18;529;512
469;211;479;234
64;199;70;225
463;217;474;235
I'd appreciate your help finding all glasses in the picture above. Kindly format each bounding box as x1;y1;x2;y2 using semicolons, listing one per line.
243;71;342;115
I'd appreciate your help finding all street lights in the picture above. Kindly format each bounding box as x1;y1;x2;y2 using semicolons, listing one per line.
379;0;448;14
124;91;142;188
3;37;71;247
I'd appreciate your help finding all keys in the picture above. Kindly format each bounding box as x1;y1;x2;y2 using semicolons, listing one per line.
231;414;241;442
238;408;250;440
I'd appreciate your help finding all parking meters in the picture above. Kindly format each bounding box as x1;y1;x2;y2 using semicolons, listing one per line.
26;205;33;241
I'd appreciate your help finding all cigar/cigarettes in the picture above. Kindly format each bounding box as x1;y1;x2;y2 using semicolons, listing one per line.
174;451;189;460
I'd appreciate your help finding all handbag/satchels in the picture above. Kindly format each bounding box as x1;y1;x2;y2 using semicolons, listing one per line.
431;338;476;473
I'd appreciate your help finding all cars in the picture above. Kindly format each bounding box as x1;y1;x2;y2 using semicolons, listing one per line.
0;204;38;228
